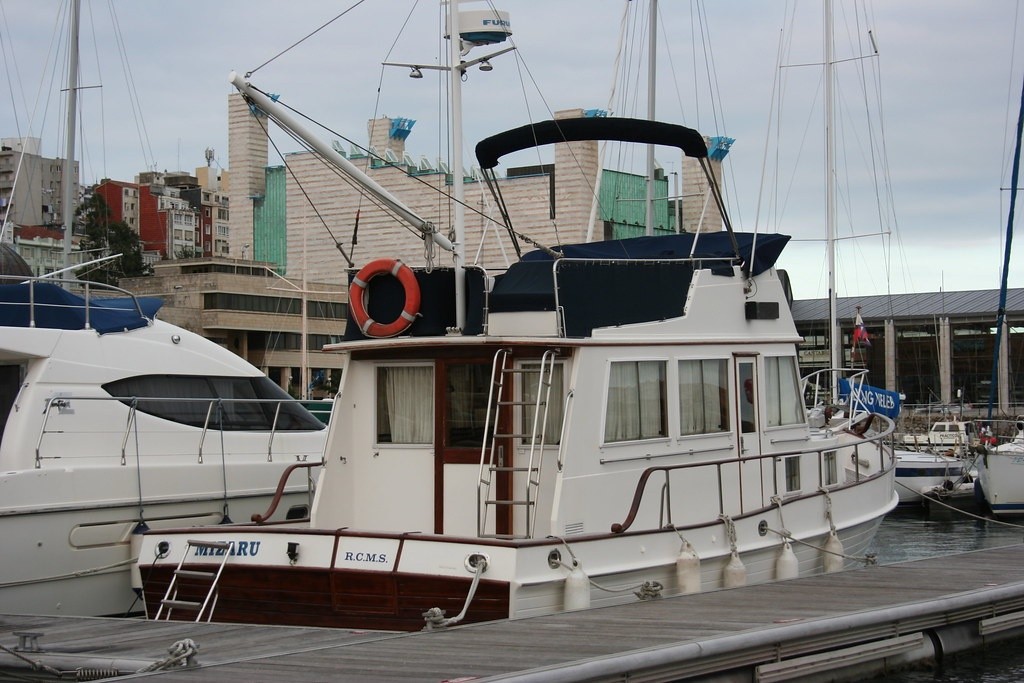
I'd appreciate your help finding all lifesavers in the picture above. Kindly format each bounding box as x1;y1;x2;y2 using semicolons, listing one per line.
347;258;422;340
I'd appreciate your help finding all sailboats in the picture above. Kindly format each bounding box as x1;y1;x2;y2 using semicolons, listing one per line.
135;3;900;637
754;4;975;505
1;0;332;628
969;1;1023;518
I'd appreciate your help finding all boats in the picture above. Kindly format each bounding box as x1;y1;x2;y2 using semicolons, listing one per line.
904;420;980;456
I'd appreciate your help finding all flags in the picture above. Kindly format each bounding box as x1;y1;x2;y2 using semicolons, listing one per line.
853;314;871;346
